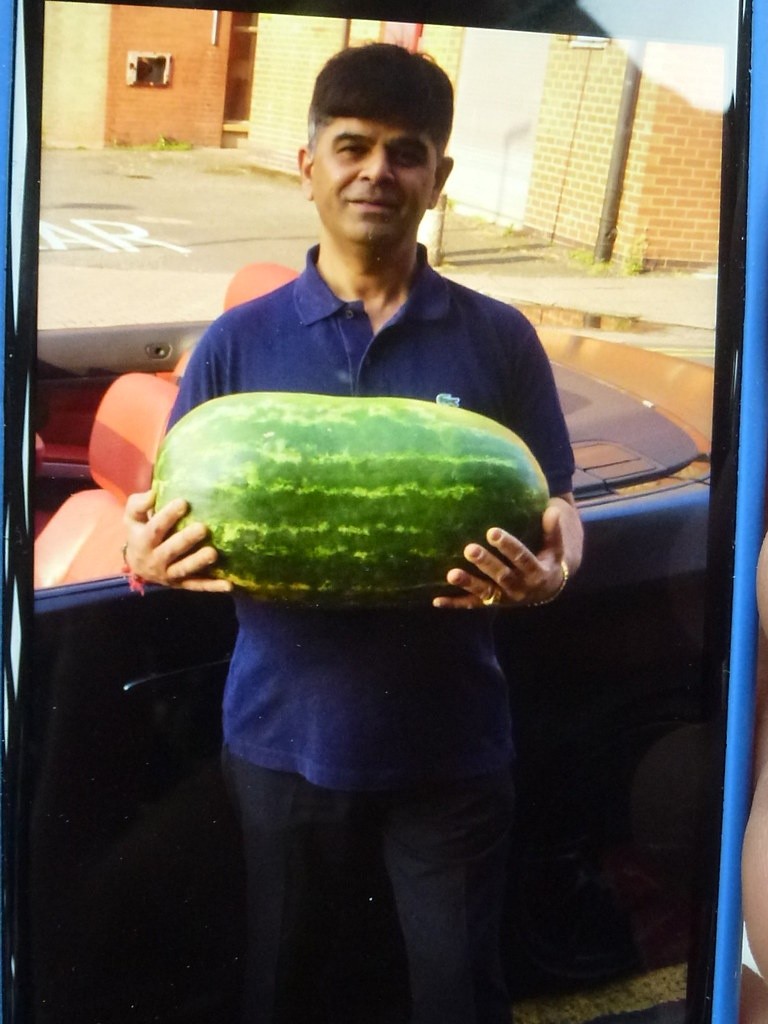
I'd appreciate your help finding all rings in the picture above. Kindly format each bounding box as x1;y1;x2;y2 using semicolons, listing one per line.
483;590;501;607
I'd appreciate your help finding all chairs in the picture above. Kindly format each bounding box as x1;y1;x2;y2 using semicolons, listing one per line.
33;263;304;589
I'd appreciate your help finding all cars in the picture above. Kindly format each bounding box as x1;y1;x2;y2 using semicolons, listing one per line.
34;300;715;1023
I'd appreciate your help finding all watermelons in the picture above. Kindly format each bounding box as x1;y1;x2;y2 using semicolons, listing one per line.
152;391;550;603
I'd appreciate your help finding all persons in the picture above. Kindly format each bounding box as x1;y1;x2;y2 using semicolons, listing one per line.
120;44;587;997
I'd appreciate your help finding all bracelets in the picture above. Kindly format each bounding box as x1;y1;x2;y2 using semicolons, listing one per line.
121;542;150;596
529;561;568;609
